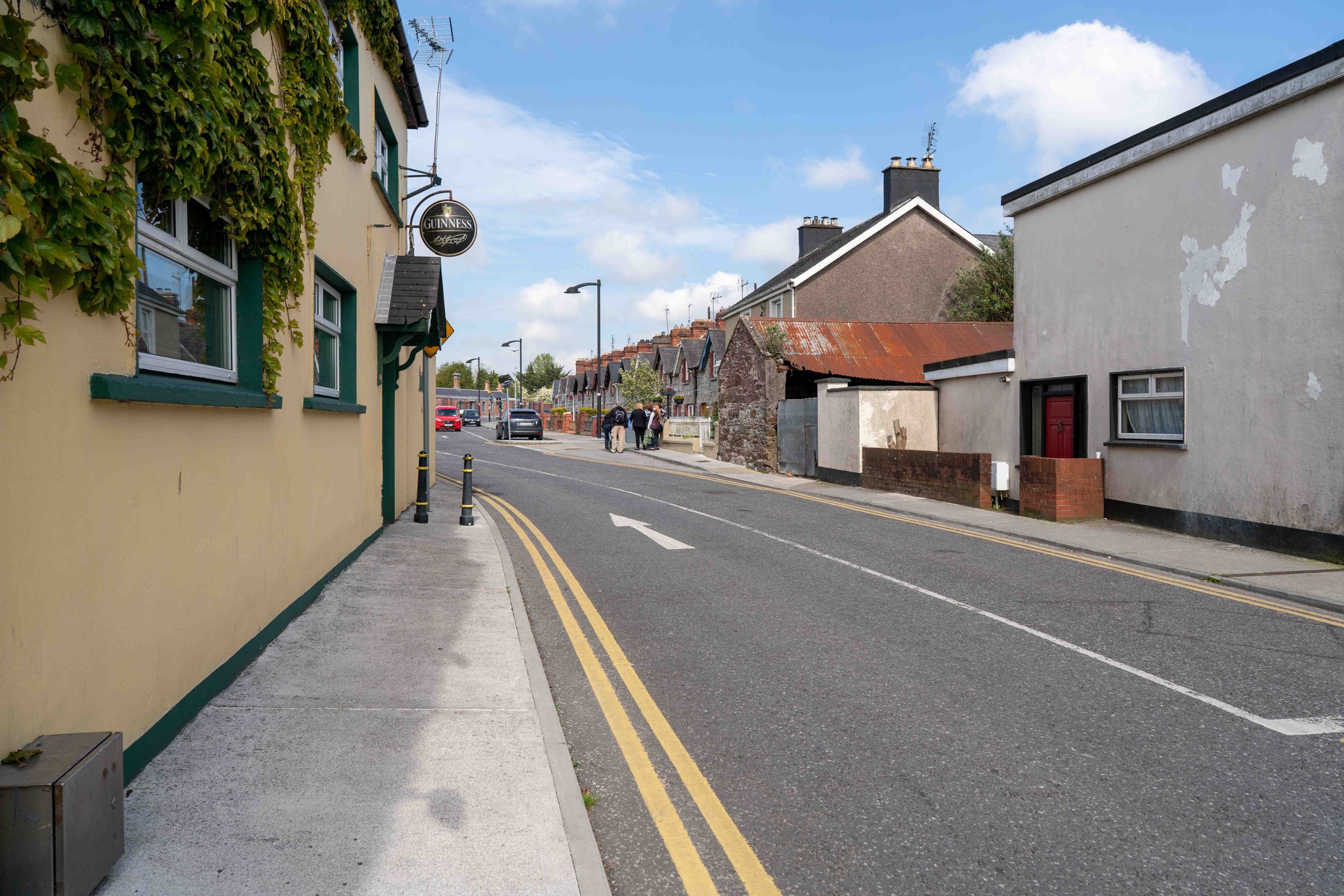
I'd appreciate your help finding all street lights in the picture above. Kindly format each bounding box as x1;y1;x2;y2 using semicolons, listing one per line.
514;366;518;409
465;356;480;416
564;279;602;438
501;338;522;408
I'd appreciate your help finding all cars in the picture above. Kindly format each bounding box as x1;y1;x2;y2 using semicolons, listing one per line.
495;407;543;440
501;380;511;387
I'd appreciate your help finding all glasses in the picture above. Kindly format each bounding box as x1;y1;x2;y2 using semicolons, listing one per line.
652;407;655;408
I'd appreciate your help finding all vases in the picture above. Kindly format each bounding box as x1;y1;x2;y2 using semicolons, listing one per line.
653;400;662;404
674;399;684;404
662;391;675;395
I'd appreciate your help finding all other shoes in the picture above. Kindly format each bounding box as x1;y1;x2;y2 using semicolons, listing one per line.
649;448;656;451
619;451;623;453
656;447;660;450
605;449;608;452
610;451;615;453
634;447;639;450
643;447;647;450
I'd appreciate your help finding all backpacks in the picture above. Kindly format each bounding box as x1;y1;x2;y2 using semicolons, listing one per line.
613;408;625;424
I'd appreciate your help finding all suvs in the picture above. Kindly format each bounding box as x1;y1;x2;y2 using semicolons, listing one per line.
462;409;481;426
436;405;461;431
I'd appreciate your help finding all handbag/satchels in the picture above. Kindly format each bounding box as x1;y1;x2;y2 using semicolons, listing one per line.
631;419;634;430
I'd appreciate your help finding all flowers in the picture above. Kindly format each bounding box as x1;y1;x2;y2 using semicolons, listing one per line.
673;394;684;400
653;396;663;400
662;386;674;391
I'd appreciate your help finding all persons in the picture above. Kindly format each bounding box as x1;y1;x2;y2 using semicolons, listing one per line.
601;401;668;454
501;408;503;412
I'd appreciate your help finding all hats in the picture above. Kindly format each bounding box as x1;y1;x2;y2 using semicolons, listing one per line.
615;401;622;406
